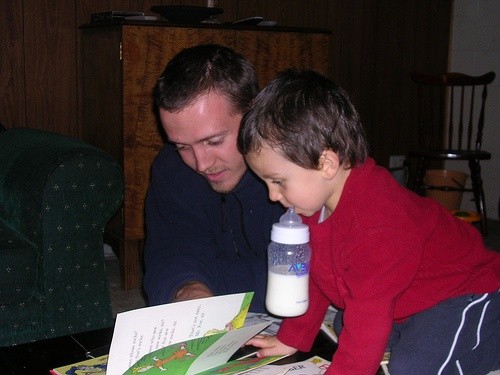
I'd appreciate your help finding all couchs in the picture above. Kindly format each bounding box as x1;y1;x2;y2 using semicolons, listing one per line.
0;128;125;348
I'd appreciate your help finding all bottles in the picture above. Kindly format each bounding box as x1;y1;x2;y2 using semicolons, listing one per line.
265;207;311;318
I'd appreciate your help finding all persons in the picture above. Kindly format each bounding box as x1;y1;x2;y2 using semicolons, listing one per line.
244;65;500;375
141;43;296;315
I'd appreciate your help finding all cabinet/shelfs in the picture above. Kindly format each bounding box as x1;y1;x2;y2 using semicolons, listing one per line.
79;23;333;288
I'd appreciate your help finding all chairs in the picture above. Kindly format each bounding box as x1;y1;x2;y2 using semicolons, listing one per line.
406;70;497;238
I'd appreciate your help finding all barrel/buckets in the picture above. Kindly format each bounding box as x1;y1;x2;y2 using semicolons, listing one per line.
424;168;466;212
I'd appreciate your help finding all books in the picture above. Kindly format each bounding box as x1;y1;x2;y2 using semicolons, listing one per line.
49;291;291;375
